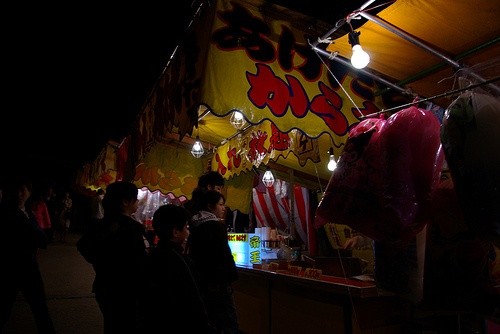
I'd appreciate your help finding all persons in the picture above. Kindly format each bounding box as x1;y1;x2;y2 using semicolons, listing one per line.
74;171;240;334
0;178;72;334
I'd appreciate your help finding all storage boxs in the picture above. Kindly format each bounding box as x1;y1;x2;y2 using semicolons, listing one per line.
229;233;259;269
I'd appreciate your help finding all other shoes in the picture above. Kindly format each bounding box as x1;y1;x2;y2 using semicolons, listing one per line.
59;240;67;243
48;239;54;244
41;247;47;250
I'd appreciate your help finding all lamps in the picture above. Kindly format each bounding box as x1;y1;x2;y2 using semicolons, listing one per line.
262;167;275;188
190;127;204;159
229;111;246;130
